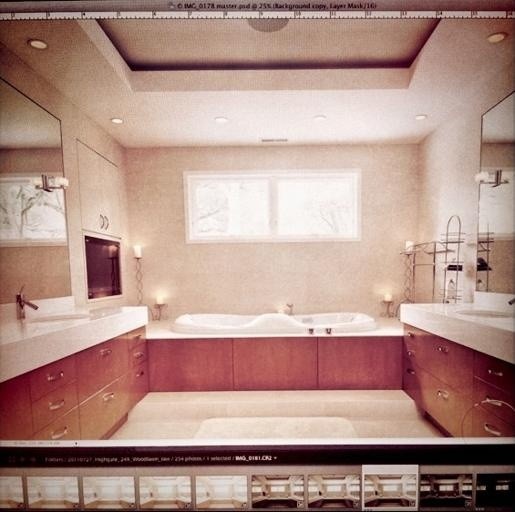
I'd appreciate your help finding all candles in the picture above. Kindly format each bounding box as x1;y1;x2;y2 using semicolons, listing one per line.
384;295;393;302
405;241;414;252
157;296;164;304
134;245;143;258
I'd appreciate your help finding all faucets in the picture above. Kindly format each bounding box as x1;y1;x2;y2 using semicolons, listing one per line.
508;298;515;305
15;282;39;319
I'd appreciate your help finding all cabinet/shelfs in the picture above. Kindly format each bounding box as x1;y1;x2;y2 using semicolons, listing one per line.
402;323;515;438
2;325;150;441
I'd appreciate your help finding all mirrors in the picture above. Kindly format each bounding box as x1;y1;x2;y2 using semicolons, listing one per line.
474;91;515;294
1;79;69;251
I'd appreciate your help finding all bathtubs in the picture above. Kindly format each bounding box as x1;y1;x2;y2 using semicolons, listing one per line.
175;312;376;335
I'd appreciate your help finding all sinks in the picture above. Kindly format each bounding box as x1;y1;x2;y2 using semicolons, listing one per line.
0;314;91;344
455;310;515;319
